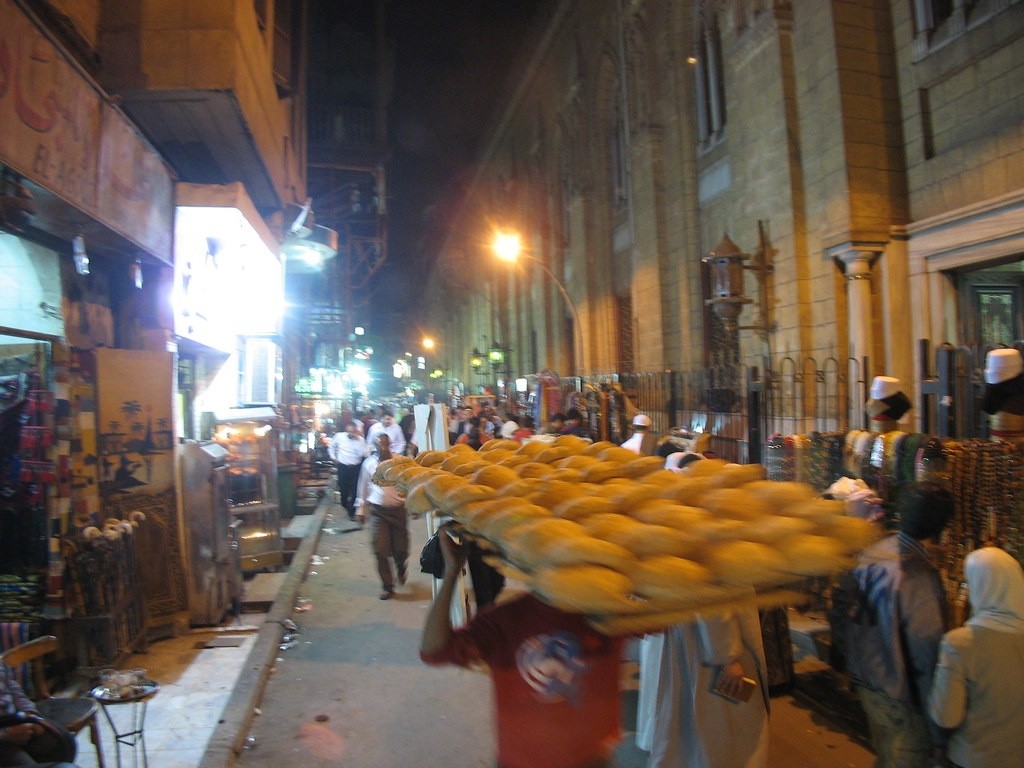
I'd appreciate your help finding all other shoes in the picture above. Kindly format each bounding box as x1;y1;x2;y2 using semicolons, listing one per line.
379;588;394;600
398;568;409;586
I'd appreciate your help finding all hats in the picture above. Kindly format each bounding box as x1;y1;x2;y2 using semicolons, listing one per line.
633;414;651;427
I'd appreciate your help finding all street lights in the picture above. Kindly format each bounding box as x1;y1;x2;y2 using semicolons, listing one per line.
491;228;585;377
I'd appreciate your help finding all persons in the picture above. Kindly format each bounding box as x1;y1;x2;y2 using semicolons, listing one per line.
513;416;532;441
820;475;924;768
923;546;1024;768
365;411;406;456
352;433;419;600
618;414;652;454
560;408;590;437
344;404;519;460
418;527;672;768
895;481;957;768
327;423;367;521
536;412;565;435
636;451;772;768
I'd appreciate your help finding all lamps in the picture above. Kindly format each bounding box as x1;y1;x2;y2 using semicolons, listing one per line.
466;341;515;375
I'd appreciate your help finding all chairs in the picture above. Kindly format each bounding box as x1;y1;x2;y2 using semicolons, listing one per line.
0;635;103;768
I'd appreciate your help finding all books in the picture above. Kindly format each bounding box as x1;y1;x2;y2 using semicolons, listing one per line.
707;668;756;706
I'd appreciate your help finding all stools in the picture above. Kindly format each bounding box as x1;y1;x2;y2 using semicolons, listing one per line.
91;681;161;767
